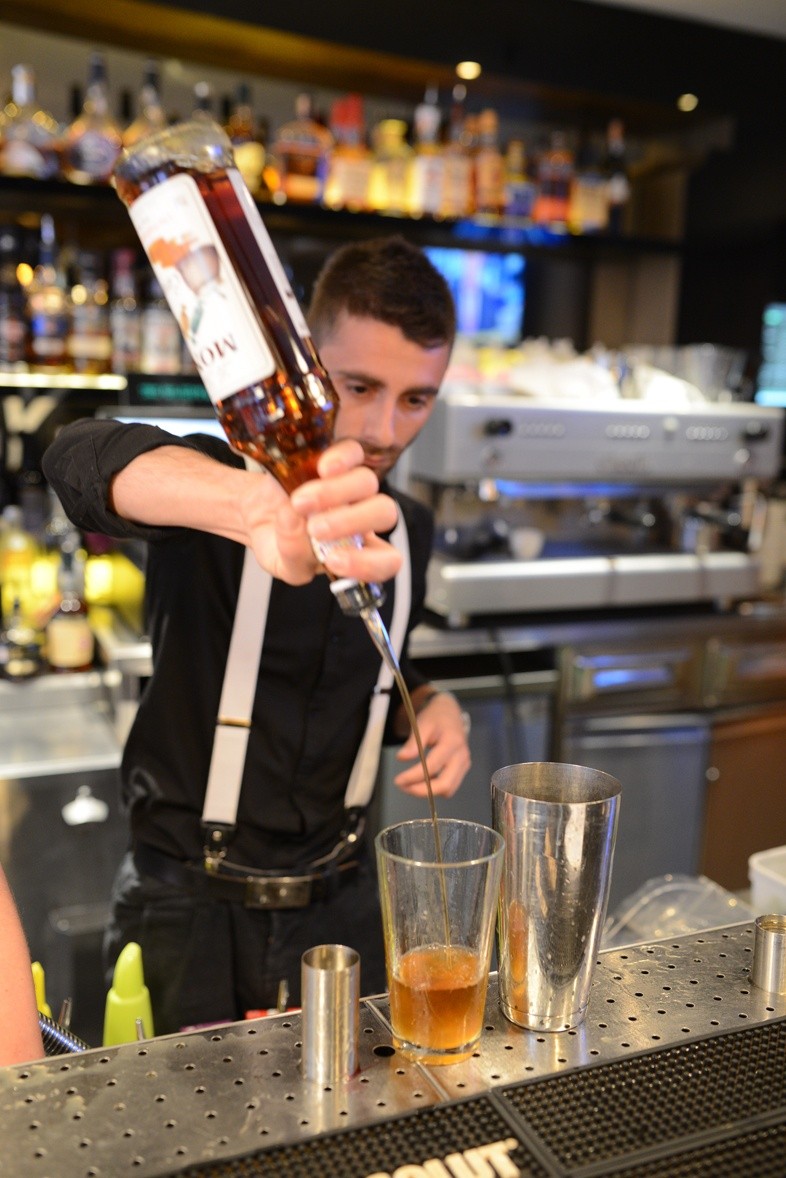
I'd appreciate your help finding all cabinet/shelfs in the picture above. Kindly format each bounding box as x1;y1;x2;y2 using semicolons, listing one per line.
0;0;684;417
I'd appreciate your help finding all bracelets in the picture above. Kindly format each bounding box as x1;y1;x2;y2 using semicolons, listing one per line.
419;691;476;732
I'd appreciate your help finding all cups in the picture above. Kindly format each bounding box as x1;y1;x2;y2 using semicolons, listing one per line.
750;913;786;994
374;817;506;1065
301;944;360;1084
490;761;623;1032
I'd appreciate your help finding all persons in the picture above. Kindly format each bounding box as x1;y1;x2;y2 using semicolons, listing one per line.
0;866;46;1067
41;234;471;1049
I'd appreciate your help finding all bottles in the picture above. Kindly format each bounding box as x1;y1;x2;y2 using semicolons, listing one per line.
268;95;377;211
113;245;210;407
124;62;266;199
475;107;535;228
0;61;124;183
113;122;399;676
0;473;94;682
539;120;632;239
0;214;110;376
375;86;475;218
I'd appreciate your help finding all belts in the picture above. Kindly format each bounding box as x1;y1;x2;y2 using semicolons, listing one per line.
128;817;367;909
